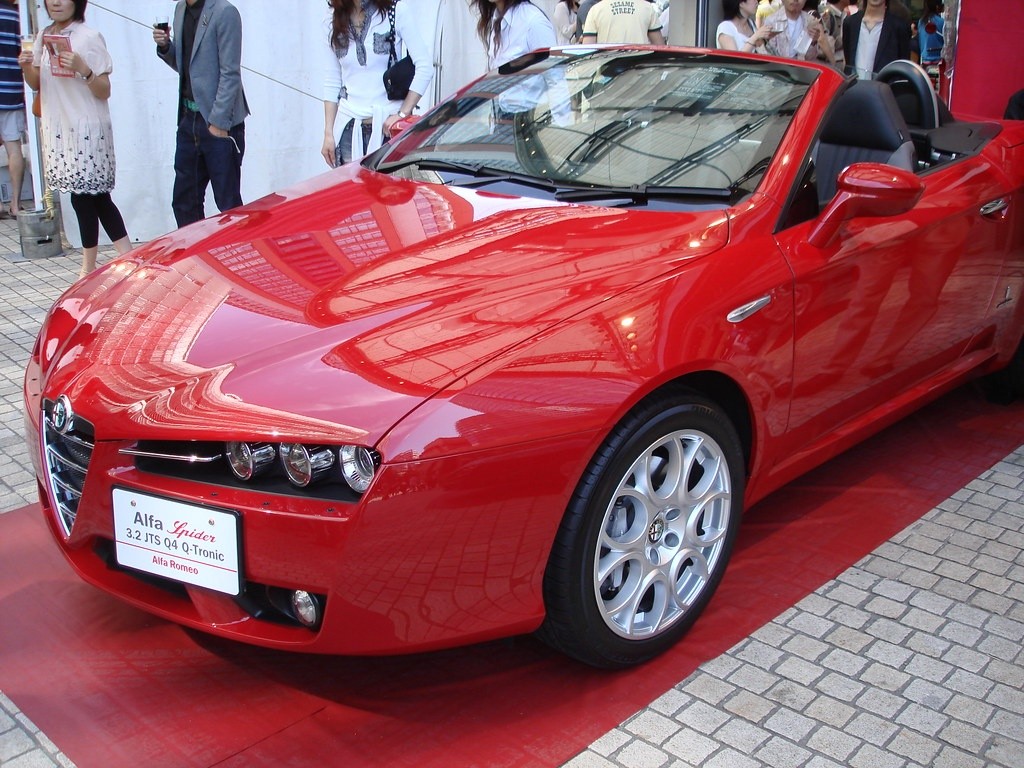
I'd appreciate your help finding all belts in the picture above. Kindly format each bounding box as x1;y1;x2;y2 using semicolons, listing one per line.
182;98;200;112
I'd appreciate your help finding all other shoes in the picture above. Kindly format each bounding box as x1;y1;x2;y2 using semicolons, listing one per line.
10;206;24;220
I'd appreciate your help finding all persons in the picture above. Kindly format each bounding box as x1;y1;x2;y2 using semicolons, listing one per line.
804;0;858;71
0;0;27;220
152;0;250;230
316;0;435;167
842;1;910;90
463;0;575;142
762;0;821;62
715;0;783;55
917;0;944;68
552;0;669;46
13;0;133;284
909;21;921;64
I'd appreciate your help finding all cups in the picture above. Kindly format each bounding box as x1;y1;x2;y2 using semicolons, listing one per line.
20;35;34;52
154;16;169;34
770;17;786;31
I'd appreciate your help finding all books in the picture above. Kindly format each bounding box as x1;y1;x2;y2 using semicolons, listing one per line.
43;33;76;78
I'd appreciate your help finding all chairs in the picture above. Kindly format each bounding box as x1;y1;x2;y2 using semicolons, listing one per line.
876;60;952;136
810;80;914;210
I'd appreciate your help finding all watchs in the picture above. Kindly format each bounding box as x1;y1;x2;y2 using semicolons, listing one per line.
82;70;92;81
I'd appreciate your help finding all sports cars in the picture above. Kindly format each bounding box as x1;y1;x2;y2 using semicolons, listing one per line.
22;43;1024;673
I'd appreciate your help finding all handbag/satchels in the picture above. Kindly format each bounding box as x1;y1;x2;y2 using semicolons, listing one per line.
32;26;50;117
382;0;415;100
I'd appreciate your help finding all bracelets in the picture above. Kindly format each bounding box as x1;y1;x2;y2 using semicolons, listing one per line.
745;38;756;46
87;73;96;85
398;111;406;118
812;41;818;46
